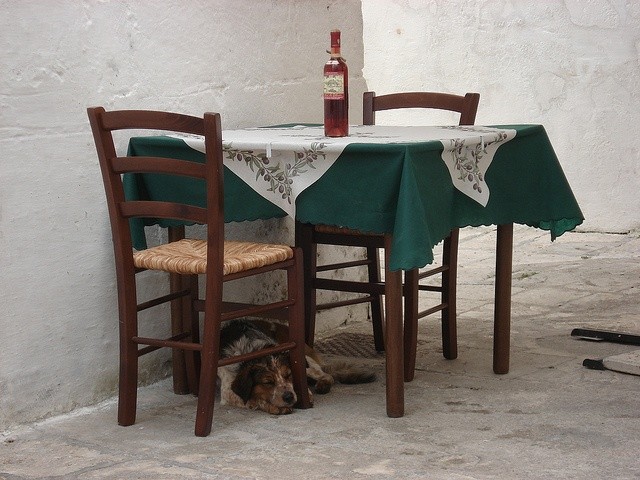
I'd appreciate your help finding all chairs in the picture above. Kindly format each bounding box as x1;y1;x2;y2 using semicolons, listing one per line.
302;90;480;383
86;105;313;437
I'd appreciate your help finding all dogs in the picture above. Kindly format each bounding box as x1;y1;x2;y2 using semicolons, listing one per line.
215;317;378;416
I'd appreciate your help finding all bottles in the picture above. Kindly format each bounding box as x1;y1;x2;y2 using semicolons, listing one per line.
323;28;349;138
